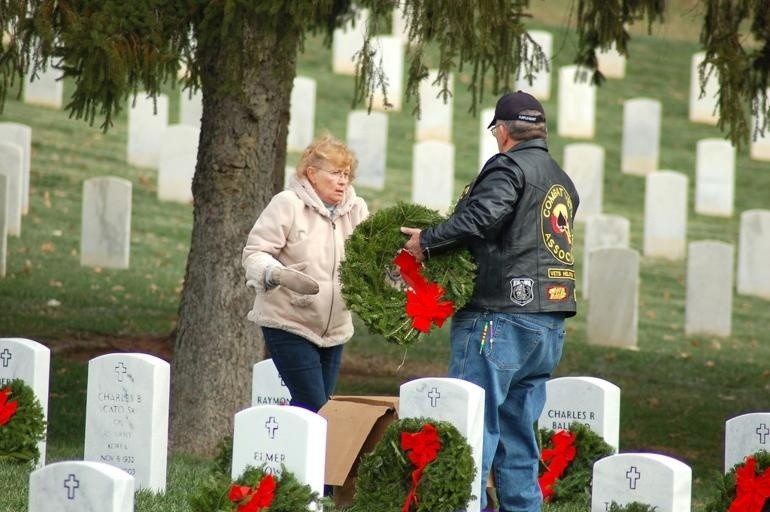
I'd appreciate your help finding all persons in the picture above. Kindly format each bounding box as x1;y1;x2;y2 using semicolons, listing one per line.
401;90;580;511
241;137;371;500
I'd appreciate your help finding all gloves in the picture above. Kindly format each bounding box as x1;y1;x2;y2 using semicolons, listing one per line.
270;262;320;298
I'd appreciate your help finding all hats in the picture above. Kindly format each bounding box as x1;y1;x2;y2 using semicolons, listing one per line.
487;91;547;130
